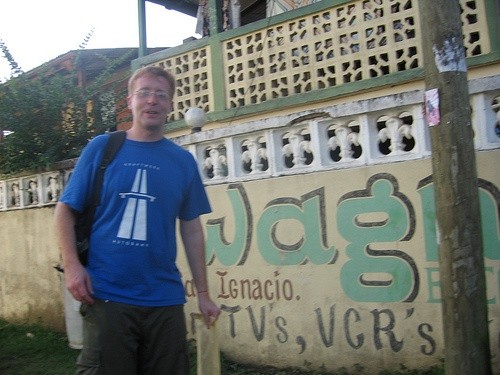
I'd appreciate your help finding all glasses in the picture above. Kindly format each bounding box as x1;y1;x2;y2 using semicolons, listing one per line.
128;89;171;101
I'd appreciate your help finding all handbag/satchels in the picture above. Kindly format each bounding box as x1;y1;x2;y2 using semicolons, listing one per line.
52;130;127;273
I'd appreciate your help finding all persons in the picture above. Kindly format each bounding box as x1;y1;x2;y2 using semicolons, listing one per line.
54;67;222;375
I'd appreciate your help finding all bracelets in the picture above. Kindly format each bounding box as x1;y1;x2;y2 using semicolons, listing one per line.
198;290;208;293
